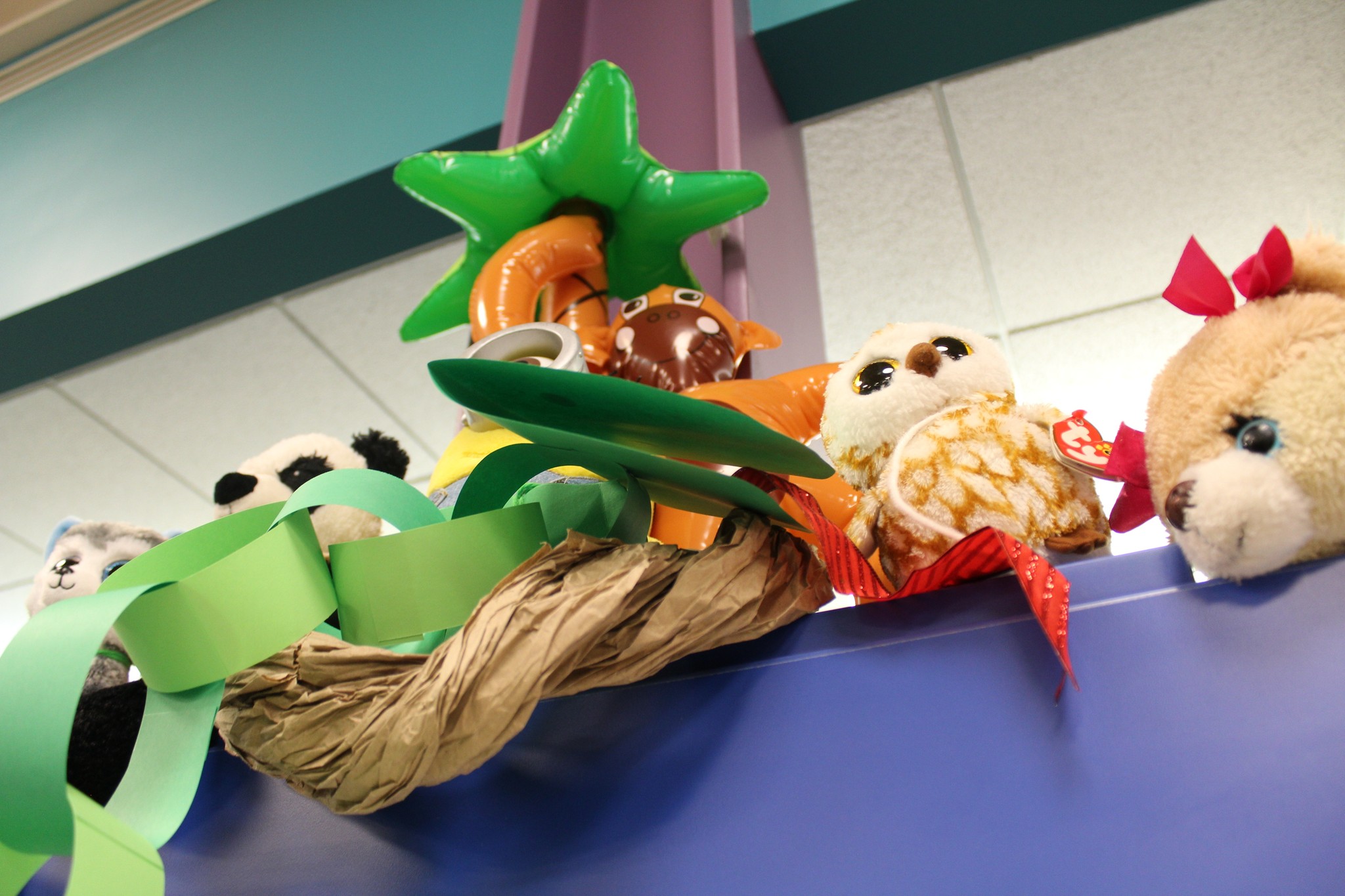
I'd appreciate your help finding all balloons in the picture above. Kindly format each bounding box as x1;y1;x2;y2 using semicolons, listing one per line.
398;61;891;590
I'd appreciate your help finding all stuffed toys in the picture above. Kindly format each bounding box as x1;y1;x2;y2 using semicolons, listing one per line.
28;426;408;695
819;227;1345;583
